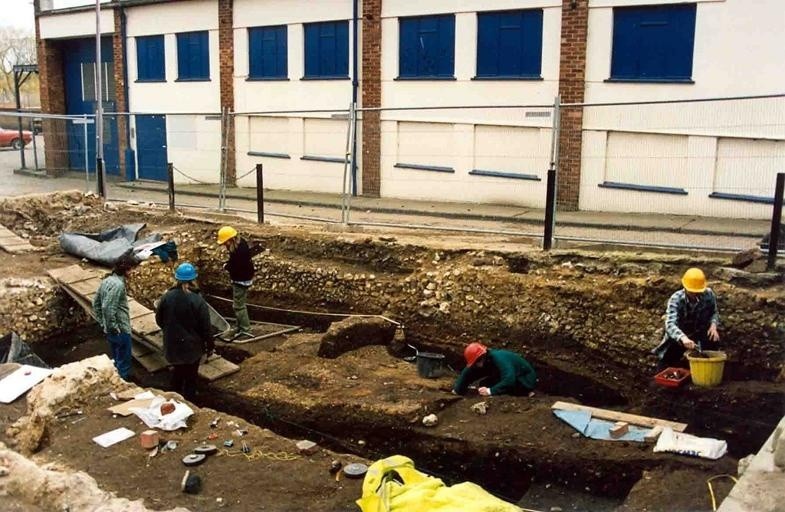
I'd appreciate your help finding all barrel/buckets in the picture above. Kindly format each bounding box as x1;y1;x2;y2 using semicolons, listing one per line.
685;350;727;388
415;351;445;380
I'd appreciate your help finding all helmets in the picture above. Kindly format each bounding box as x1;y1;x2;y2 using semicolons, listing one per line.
216;225;238;245
682;267;708;293
464;343;488;368
174;263;198;281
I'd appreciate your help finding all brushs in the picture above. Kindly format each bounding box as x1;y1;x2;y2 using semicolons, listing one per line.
57;411;83;418
181;470;201;493
210;416;220;428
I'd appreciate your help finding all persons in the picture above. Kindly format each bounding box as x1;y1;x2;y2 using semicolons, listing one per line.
154;261;215;407
651;265;722;369
217;222;256;339
453;341;539;399
92;258;133;385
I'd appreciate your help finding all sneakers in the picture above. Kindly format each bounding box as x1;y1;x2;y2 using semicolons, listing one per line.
228;327;252;338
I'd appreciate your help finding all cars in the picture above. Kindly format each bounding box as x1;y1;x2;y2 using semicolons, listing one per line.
0;127;33;150
